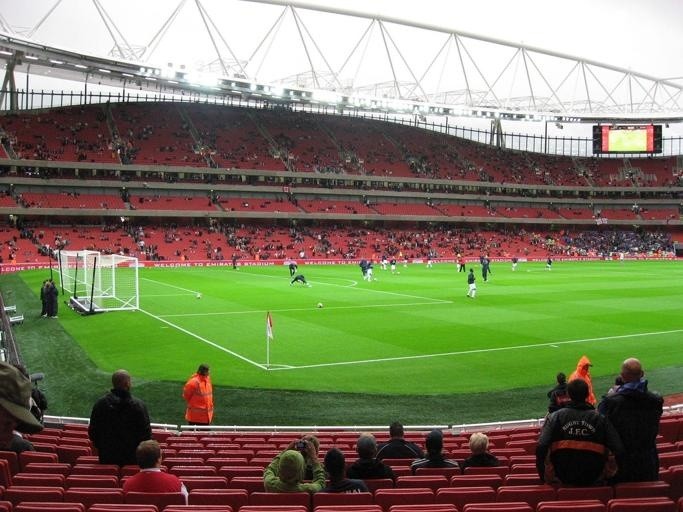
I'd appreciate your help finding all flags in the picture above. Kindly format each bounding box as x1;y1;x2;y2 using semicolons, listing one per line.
266;313;274;339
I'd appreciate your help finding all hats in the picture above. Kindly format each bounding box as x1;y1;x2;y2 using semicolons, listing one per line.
0;360;45;434
196;365;208;376
426;428;443;450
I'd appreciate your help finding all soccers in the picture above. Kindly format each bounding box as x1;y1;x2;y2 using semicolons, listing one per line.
197;295;201;298
318;304;323;307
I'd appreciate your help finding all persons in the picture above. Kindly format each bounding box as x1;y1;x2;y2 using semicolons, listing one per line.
122;440;189;505
534;356;665;490
39;279;58;317
0;101;683;298
88;369;151;466
263;421;499;495
1;362;45;452
183;365;215;432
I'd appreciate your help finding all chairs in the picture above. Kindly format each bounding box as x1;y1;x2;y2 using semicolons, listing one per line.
2;98;682;266
1;388;682;512
